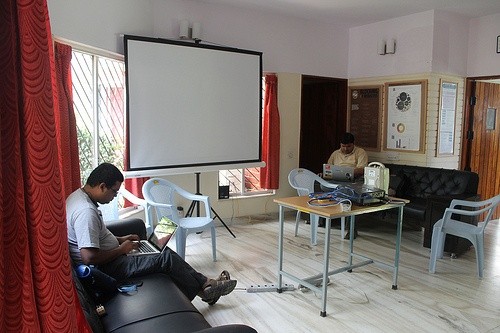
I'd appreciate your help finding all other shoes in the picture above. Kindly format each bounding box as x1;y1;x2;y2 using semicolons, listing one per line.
344;231;358;240
209;271;230;305
202;279;237;303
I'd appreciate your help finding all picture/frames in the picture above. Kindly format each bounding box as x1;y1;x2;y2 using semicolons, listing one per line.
347;85;383;153
381;79;428;154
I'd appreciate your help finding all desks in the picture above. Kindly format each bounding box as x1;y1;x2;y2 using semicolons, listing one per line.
274;196;411;317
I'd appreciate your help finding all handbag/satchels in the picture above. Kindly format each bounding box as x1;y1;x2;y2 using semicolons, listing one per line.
75;265;116;305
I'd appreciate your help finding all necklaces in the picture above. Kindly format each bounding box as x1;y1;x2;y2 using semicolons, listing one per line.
82;187;99;207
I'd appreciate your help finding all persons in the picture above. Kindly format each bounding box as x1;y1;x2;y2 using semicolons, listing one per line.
327;133;369;239
65;162;236;306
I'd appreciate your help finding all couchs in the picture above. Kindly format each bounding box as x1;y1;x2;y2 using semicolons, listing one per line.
301;162;481;259
71;217;257;333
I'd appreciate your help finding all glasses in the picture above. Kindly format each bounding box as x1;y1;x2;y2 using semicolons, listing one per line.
100;181;118;196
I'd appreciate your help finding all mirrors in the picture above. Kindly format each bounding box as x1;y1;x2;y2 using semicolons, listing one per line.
435;78;459;158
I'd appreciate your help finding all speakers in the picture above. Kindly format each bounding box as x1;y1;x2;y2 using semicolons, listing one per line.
363;166;389;196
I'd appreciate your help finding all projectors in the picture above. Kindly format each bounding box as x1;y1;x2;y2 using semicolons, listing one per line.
336;182;386;204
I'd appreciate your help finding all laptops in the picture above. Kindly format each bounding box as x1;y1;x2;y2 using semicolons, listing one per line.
323;164;354;183
125;216;179;256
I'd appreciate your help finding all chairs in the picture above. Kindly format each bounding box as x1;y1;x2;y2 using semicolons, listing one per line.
142;178;216;262
428;195;500;278
287;168;345;246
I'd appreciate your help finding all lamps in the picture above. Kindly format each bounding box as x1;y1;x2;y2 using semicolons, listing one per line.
179;20;202;41
377;40;395;55
